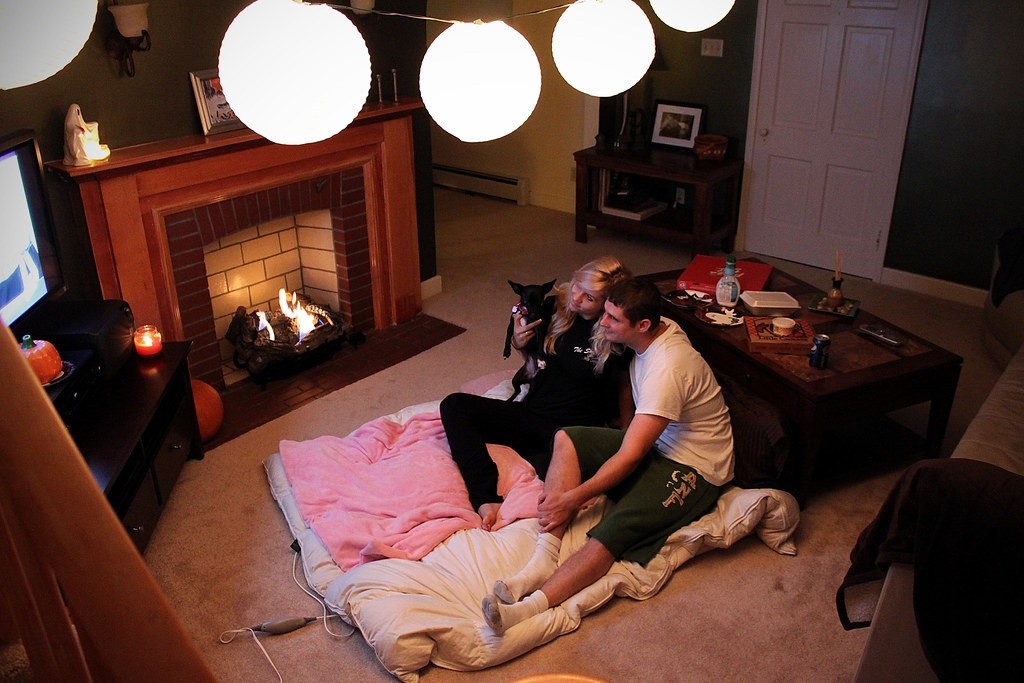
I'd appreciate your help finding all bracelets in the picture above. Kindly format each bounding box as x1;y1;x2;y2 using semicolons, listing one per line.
511;335;522;350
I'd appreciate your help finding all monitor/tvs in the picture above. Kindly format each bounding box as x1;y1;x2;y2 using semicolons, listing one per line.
0;128;68;333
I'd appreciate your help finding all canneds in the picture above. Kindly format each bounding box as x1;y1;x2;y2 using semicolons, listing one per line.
809;334;831;370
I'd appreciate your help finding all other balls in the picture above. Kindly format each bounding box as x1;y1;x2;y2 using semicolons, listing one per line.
189;379;224;444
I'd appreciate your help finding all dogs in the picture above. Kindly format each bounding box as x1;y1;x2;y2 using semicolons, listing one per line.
501;279;560;402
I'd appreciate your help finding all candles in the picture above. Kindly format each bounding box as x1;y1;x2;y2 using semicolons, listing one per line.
135;322;162;358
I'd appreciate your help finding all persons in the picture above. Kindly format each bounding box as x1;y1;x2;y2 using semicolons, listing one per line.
440;256;634;532
482;275;736;635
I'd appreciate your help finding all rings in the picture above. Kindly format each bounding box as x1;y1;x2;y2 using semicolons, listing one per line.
526;332;529;336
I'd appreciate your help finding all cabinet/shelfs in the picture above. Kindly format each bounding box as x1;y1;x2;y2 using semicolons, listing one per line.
59;335;211;553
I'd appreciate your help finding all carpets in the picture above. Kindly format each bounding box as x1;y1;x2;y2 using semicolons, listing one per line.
146;182;1024;683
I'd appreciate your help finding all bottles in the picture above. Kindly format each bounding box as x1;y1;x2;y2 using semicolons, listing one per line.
715;255;740;307
134;326;163;356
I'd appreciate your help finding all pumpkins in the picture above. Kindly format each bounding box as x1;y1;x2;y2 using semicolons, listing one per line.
18;334;63;385
191;379;223;442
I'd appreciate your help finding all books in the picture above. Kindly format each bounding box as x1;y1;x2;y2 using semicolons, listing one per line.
598;168;611;211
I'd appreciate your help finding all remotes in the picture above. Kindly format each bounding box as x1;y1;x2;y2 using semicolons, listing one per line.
859;324;904;346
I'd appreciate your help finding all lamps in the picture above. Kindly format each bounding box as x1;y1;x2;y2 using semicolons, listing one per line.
551;0;658;99
416;16;544;146
214;0;377;146
104;2;154;82
649;0;736;34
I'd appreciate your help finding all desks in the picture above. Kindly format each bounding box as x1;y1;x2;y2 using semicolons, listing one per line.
571;143;744;263
628;253;966;494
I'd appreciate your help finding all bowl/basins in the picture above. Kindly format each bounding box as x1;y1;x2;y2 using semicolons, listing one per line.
739;290;801;316
772;317;796;336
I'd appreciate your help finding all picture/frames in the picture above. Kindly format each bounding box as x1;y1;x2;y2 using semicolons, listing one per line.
643;99;711;153
188;66;247;138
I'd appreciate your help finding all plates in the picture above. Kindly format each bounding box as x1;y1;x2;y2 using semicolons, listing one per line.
664;289;715;309
807;293;861;318
859;323;904;346
694;308;745;327
